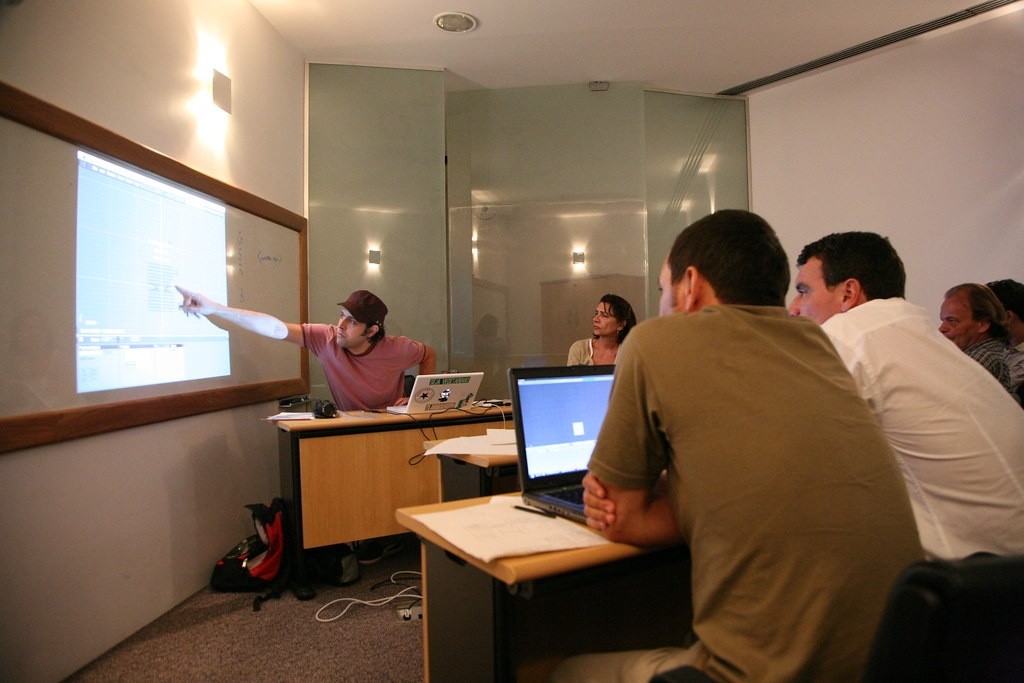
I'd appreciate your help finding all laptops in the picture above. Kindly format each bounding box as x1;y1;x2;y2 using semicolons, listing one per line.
387;372;484;414
507;363;616;525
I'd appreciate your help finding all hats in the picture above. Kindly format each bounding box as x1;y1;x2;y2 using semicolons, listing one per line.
337;290;388;330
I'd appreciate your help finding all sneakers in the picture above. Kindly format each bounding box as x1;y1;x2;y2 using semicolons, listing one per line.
357;540;402;563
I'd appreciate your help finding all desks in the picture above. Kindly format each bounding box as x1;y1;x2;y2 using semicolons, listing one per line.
424;439;521;505
266;401;518;601
396;491;695;683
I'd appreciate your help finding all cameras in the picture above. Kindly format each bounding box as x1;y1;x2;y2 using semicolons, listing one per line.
313;400;337;419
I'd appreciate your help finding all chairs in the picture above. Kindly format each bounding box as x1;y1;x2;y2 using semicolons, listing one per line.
649;551;1024;683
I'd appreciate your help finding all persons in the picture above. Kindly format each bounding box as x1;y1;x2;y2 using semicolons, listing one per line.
548;209;921;683
175;286;437;564
567;294;637;366
788;231;1024;557
987;280;1024;404
939;284;1012;393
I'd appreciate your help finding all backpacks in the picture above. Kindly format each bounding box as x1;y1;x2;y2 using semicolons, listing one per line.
211;497;286;591
